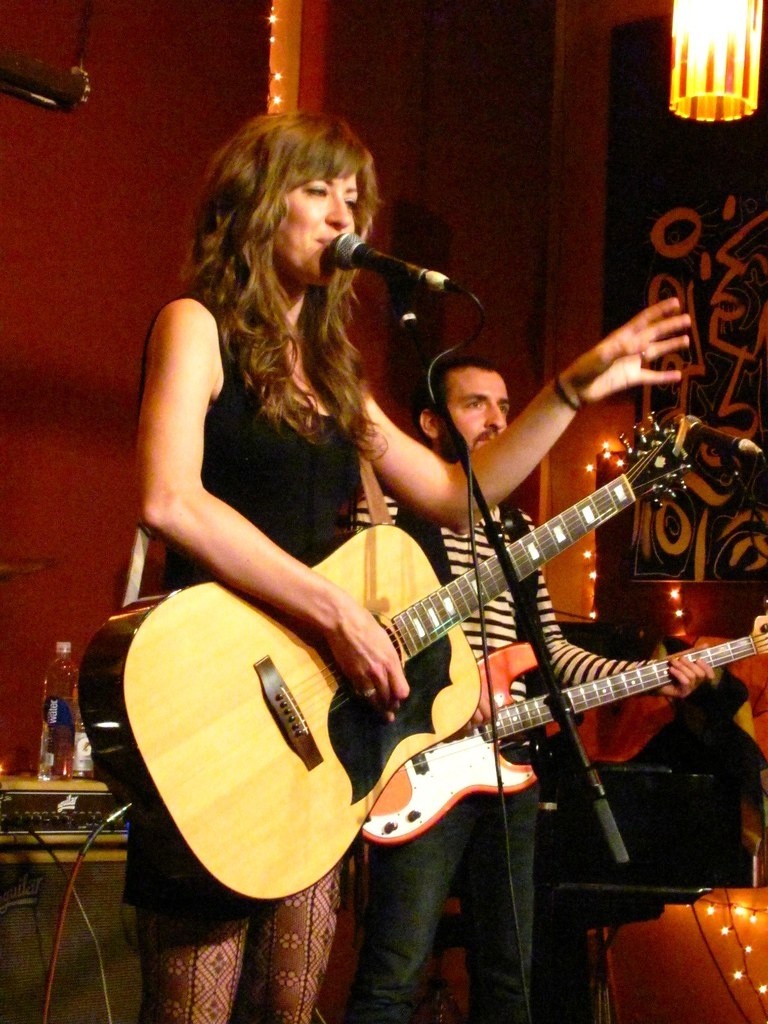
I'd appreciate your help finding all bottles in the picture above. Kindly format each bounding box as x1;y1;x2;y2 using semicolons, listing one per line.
413;978;462;1024
71;693;93;778
38;642;78;780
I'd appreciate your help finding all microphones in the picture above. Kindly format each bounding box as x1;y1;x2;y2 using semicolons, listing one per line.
683;415;764;455
329;232;463;293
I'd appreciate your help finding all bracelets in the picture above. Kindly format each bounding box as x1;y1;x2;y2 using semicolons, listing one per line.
553;374;582;411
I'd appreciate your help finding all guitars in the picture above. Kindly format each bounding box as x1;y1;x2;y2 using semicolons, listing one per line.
76;409;697;914
361;597;768;849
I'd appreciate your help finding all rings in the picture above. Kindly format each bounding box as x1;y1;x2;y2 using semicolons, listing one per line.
641;352;646;365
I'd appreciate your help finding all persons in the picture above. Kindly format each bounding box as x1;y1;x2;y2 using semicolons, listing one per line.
340;353;715;1024
121;113;694;1024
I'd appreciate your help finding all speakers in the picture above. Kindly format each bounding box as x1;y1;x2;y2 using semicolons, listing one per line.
0;847;145;1024
